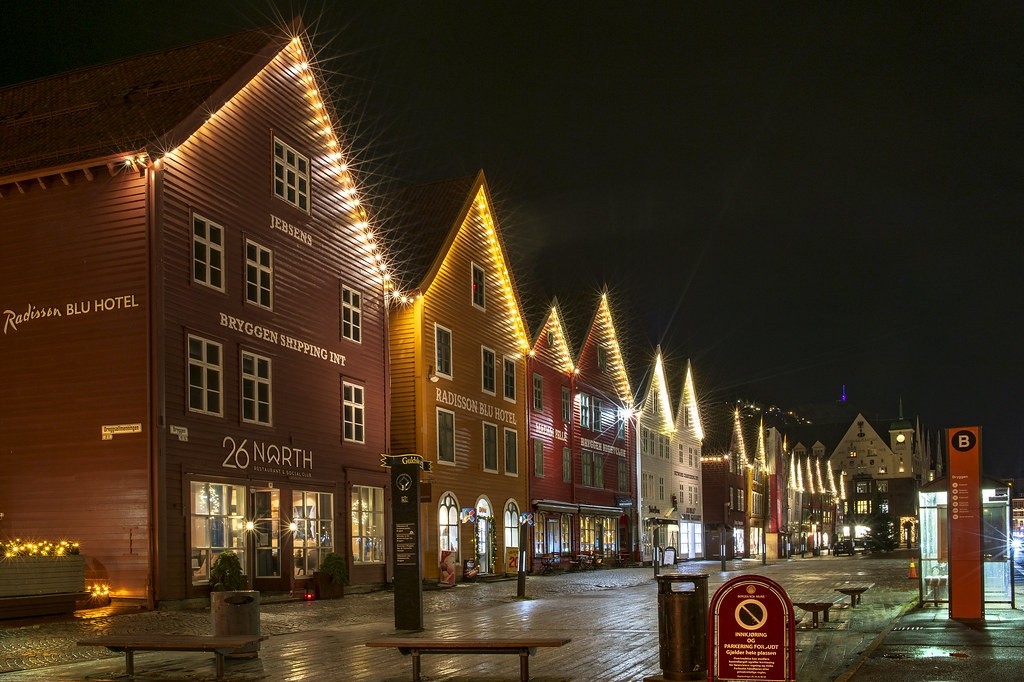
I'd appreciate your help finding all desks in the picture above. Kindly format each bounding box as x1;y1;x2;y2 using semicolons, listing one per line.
616;554;631;568
536;556;558;575
577;554;588;571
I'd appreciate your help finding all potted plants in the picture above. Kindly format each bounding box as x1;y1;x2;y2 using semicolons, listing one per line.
311;551;350;601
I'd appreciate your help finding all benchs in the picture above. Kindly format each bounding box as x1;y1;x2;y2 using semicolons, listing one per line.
77;635;269;678
364;638;571;682
793;593;849;629
834;583;875;607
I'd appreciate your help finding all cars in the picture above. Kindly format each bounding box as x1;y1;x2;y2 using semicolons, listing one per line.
833;541;855;556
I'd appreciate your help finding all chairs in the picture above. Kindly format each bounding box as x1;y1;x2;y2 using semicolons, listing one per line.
539;552;561;576
616;557;631;568
568;551;605;571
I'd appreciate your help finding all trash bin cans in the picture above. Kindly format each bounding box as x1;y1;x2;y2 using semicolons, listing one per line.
210;590;261;659
655;573;712;682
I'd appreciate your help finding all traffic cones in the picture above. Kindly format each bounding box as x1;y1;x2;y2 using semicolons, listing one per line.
906;558;919;579
